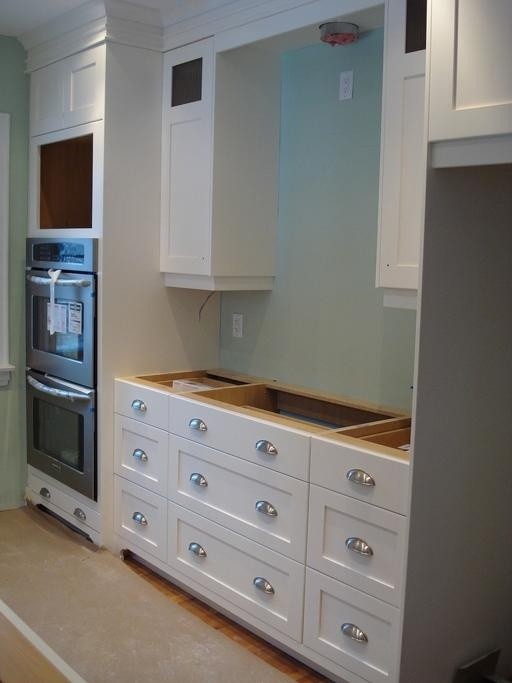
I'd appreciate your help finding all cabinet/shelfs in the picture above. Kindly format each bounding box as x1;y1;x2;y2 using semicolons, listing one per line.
28;43;162;237
300;438;413;683
374;0;426;310
427;0;512;170
113;379;167;582
167;391;311;667
159;38;281;292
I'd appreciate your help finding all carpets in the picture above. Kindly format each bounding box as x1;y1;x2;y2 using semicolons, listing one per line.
0;508;297;683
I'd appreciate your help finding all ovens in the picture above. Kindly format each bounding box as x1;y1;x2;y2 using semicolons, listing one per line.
26;238;98;389
26;367;97;502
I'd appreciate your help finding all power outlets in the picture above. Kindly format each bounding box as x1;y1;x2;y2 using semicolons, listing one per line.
232;314;242;338
339;71;353;99
